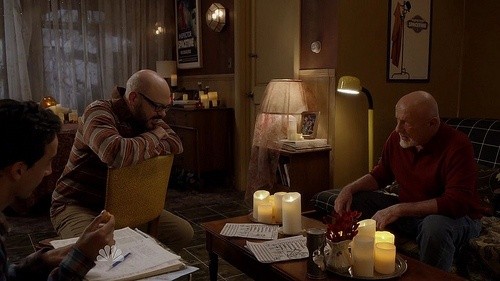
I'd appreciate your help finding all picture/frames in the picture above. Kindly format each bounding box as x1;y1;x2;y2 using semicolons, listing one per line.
385;0;432;83
175;0;203;69
301;111;321;139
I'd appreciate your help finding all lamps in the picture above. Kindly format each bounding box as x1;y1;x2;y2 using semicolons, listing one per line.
337;75;375;174
205;2;228;32
253;77;309;149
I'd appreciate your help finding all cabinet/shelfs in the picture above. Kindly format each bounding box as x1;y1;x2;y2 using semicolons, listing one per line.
171;106;235;183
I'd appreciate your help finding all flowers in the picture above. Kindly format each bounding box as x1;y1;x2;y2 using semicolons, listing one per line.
325;207;362;243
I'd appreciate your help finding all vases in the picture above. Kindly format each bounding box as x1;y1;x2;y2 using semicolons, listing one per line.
326;239;353;273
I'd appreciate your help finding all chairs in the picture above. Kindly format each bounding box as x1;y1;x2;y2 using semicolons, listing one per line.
34;152;175;252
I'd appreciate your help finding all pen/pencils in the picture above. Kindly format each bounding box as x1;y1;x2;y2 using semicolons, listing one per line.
106;252;131;272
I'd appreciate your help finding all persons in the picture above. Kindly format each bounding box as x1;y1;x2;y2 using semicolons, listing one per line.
51;69;195;254
336;91;484;271
0;97;115;281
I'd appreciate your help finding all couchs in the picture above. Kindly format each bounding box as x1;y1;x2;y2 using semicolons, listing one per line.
315;115;500;281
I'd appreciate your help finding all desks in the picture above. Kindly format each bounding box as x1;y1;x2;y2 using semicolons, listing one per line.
197;206;467;281
252;143;332;216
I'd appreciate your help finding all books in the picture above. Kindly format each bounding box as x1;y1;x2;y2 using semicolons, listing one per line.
52;225;187;281
273;141;330;151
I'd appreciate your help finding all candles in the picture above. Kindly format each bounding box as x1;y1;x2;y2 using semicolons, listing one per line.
350;218;397;277
170;73;218;109
248;183;302;235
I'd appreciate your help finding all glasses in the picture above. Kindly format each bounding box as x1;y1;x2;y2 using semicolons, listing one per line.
137;91;172;113
